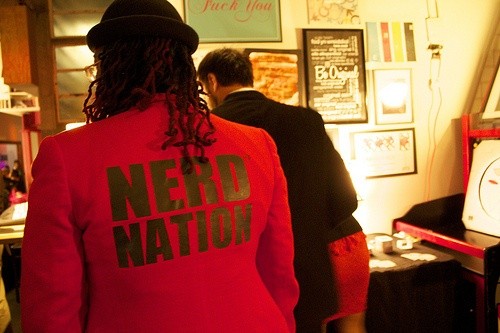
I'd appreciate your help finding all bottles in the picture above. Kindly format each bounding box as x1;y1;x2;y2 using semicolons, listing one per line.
0;77;10;99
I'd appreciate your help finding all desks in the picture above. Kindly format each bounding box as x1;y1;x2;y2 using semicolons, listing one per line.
364;232;459;333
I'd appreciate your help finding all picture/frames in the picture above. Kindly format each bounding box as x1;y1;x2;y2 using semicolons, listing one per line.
302;27;368;125
244;48;304;106
183;0;283;44
349;128;418;180
373;69;414;125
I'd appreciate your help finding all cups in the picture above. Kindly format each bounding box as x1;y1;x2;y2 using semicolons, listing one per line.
375;235;393;252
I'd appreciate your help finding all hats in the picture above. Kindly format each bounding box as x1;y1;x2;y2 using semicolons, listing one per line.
86;0;199;56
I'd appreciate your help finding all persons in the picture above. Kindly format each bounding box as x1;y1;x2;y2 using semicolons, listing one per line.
0;160;26;210
20;0;301;333
320;215;369;333
198;49;357;333
